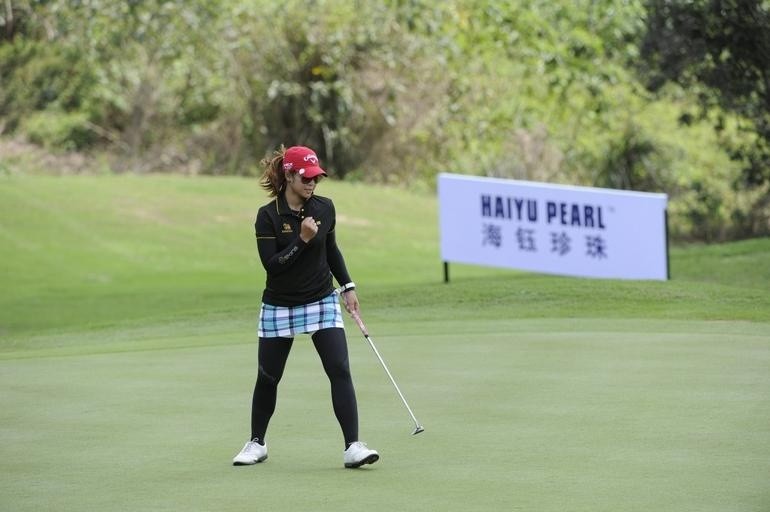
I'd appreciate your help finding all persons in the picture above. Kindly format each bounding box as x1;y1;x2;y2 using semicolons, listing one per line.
222;142;380;469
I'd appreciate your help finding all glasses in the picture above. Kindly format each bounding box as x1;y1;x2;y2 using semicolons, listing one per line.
300;175;321;184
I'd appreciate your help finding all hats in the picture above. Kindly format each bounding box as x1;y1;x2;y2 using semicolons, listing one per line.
283;146;328;179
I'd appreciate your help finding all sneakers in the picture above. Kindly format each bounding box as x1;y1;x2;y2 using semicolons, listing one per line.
343;441;379;468
232;441;268;465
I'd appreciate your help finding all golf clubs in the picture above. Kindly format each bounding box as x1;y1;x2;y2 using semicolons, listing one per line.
339;292;424;436
